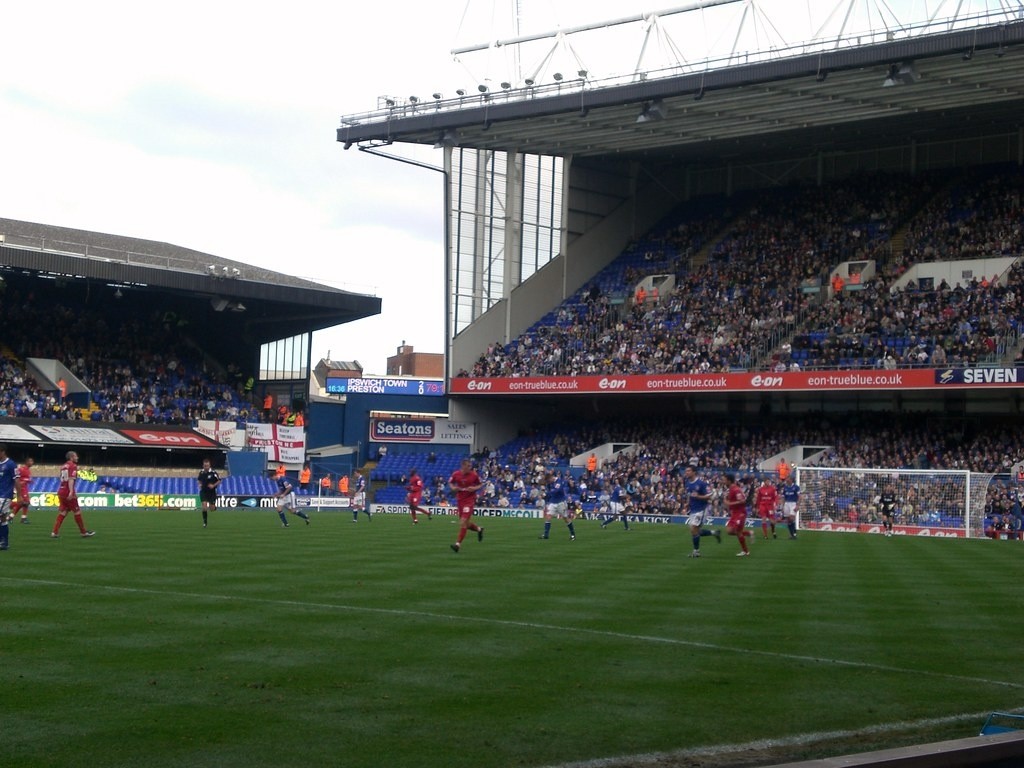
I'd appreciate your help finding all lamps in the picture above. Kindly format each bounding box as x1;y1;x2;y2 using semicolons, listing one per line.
525;79;533;86
410;96;420;103
208;264;241;278
344;143;352;150
553;73;563;83
481;122;491;130
579;107;588;117
456;89;467;96
113;282;123;298
433;93;443;99
478;85;489;93
816;72;826;82
962;49;973;60
386;99;397;106
994;43;1006;58
635;112;651;123
883;74;896;87
578;70;588;80
694;90;703;100
501;82;511;90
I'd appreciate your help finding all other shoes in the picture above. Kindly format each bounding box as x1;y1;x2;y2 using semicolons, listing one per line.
765;533;777;540
749;531;755;545
789;534;797;539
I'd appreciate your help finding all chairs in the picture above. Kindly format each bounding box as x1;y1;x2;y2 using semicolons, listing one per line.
369;420;1024;533
476;158;1024;371
12;358;258;423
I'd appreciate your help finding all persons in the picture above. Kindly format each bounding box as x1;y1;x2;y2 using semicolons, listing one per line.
5;458;34;524
268;471;310;528
756;477;779;539
685;465;721;558
448;460;484;554
880;484;898;537
538;473;576;541
783;476;800;539
50;451;96;538
400;167;1024;541
0;280;388;494
405;468;433;526
601;481;631;531
0;443;23;551
722;474;754;556
352;470;372;523
197;459;221;528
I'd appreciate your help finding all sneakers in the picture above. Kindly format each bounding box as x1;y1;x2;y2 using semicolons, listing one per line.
368;513;372;522
51;533;60;538
82;531;96;537
412;523;418;526
688;553;701;558
281;524;289;528
570;535;576;542
450;544;459;553
353;520;357;522
538;535;548;539
20;520;31;524
600;524;607;530
624;527;632;531
203;524;207;528
6;516;13;525
305;515;310;525
736;551;750;557
478;527;484;542
715;530;721;544
428;511;432;520
